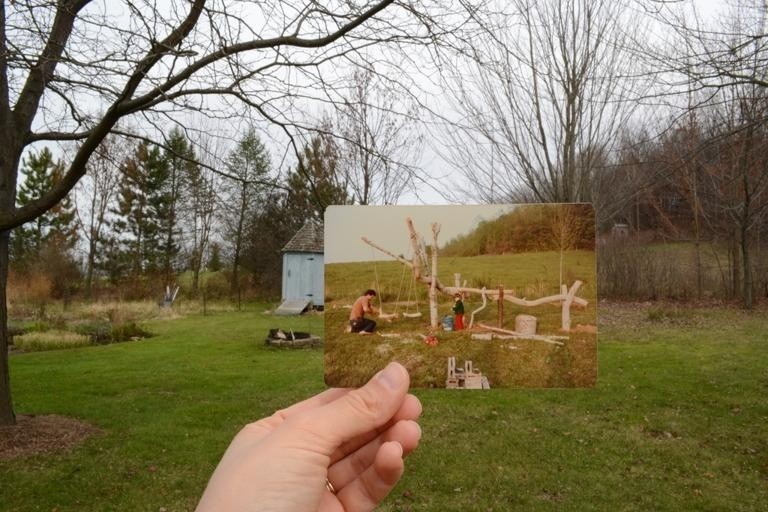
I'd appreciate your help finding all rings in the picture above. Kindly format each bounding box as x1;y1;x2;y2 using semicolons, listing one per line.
325;478;334;494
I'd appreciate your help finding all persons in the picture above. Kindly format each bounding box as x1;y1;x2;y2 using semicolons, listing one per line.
195;360;424;512
450;293;465;330
346;289;380;336
